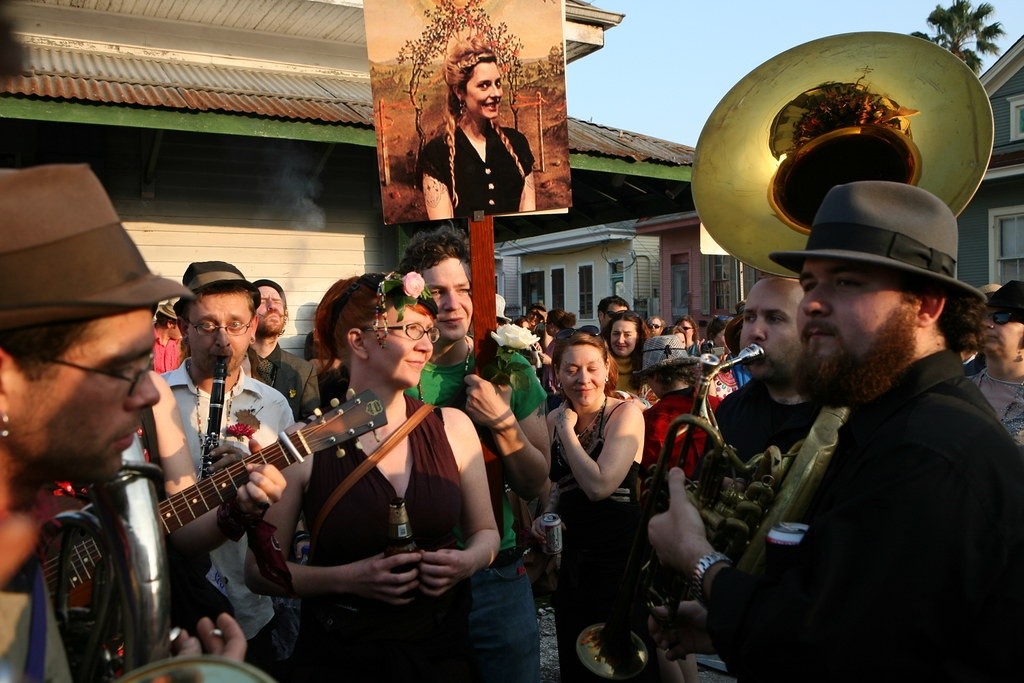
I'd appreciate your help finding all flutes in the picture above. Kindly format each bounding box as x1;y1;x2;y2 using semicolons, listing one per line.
197;357;228;484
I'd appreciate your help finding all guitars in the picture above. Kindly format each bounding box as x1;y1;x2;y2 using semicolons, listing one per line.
38;389;388;601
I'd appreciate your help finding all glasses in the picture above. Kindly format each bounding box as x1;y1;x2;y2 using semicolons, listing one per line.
604;310;617;318
682;327;694;330
357;322;441;344
556;325;601;343
186;315;255;336
982;311;1024;325
712;314;734;326
7;343;157;396
646;323;663;329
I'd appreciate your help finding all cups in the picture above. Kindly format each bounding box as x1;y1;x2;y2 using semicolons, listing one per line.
531;351;543;368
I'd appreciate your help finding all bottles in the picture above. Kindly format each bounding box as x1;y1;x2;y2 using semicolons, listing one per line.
386;497;420;600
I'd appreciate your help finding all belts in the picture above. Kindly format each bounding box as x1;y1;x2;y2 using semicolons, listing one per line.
488;547;524;569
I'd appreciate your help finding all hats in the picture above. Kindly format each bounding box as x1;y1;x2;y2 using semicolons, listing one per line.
496;293;512;322
253;280;286;309
633;334;702;376
173;261;261;317
982;280;1024;325
159;297;181;319
0;162;198;329
769;180;989;308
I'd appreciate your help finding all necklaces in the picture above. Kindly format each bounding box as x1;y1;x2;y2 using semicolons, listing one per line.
577;403;605;439
188;362;237;466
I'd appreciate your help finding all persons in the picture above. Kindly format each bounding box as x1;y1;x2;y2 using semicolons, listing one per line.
153;260;298;646
648;182;1024;683
243;270;500;683
416;34;536;220
0;136;1024;683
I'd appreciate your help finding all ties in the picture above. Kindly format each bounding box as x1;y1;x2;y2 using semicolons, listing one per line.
257;359;274;385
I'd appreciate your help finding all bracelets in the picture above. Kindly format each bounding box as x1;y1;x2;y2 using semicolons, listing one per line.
691;551;733;607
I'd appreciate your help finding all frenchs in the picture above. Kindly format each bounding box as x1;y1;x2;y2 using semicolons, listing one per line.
38;422;173;683
691;31;994;576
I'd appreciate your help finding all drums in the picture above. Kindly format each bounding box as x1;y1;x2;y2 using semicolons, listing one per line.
111;654;276;683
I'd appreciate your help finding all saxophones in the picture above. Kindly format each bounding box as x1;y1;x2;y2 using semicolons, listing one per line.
575;343;764;682
